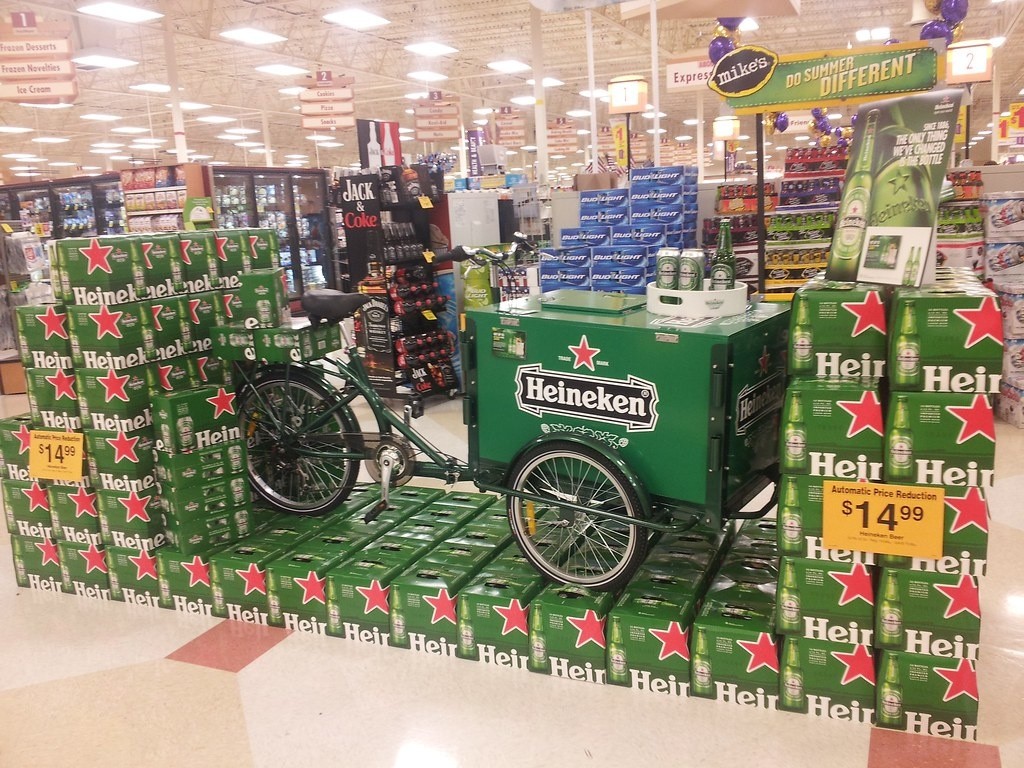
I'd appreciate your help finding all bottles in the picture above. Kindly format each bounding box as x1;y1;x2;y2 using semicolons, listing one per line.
780;477;805;556
783;637;805;712
390;585;406;646
692;625;713;695
828;108;880;282
460;596;476;659
0;231;283;628
608;615;628;685
938;170;986;234
792;293;815;375
389;282;451;362
879;652;904;731
529;605;548;672
359;262;392;371
880;570;903;648
887;396;917;484
709;218;734;291
326;576;342;635
778;559;803;633
898;299;920;389
785;389;807;475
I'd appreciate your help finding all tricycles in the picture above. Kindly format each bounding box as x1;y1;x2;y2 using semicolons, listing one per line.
234;231;794;591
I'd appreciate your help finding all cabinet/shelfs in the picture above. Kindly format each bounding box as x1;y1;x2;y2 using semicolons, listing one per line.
337;166;458;419
122;164;339;318
0;173;127;239
443;145;854;305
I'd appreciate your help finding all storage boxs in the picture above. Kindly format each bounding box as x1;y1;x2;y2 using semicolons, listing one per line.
211;483;782;708
0;229;349;619
776;172;1024;742
540;167;701;296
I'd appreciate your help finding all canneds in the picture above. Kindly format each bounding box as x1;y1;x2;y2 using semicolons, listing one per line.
678;248;705;305
870;155;935;228
656;247;681;304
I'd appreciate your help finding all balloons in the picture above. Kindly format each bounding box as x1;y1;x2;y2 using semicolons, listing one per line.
765;107;859;147
883;0;969;48
709;18;746;64
